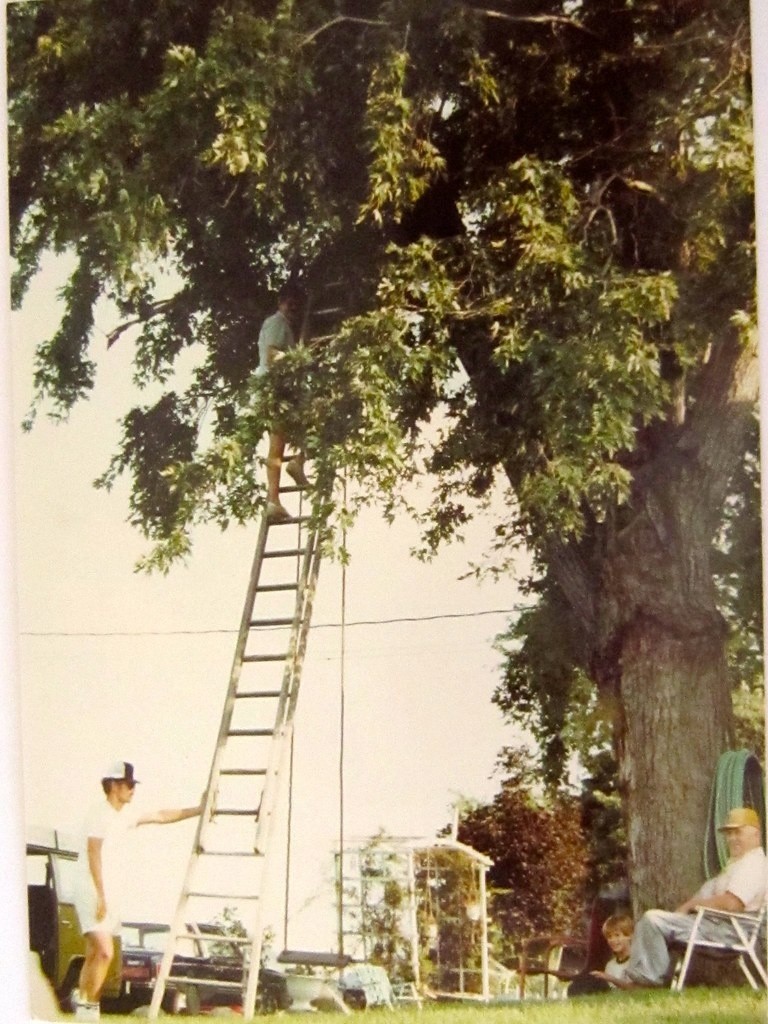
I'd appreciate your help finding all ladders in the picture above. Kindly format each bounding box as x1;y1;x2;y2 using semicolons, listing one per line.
141;271;360;1022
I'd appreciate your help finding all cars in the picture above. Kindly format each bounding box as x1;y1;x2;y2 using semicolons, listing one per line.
105;923;288;1017
26;843;123;1009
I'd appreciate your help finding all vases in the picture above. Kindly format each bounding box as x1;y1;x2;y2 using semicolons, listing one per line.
286;974;324;1012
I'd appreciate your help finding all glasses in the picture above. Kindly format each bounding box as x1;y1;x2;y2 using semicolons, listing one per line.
114;779;134;790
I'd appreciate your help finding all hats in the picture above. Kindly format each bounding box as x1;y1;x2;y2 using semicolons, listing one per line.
102;760;139;785
716;807;760;831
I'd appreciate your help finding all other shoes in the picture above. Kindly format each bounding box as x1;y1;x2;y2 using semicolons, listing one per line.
286;461;309;485
72;988;100;1019
265;507;292;523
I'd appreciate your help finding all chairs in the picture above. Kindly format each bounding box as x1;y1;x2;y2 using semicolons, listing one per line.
520;895;617;999
670;890;768;996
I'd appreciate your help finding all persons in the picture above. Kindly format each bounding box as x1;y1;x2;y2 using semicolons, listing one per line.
568;915;636;997
625;808;767;990
251;284;311;521
70;762;211;1017
